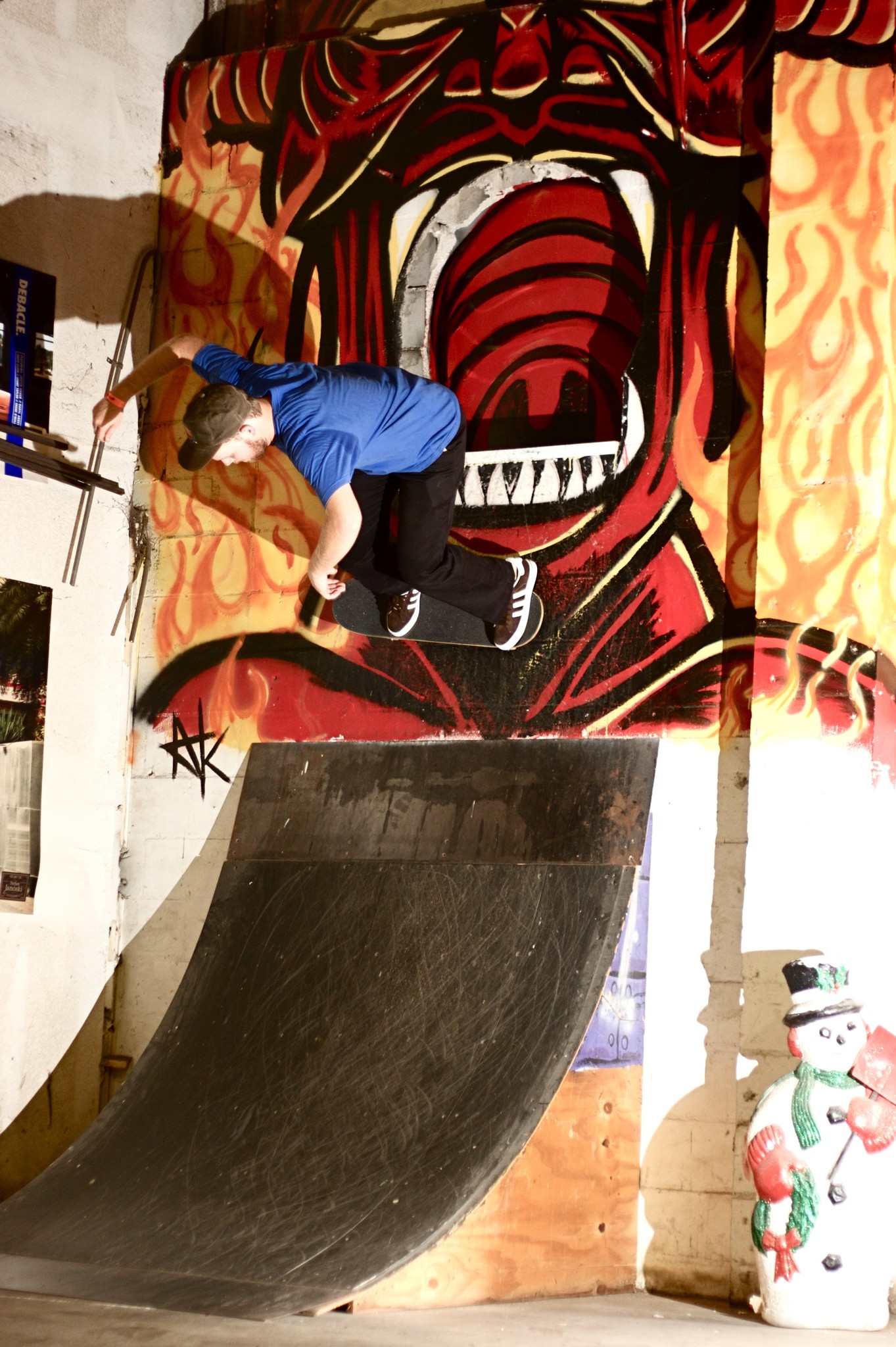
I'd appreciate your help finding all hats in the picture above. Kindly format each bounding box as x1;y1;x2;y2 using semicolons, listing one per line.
177;380;252;472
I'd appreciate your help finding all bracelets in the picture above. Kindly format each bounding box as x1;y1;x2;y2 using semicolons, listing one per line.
102;390;127;411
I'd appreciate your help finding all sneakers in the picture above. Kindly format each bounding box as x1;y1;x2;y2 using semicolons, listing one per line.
491;556;537;650
387;585;421;638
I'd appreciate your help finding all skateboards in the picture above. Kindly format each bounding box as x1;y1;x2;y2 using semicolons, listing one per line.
333;579;544;651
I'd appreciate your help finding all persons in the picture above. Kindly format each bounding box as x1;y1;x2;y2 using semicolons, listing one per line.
92;331;538;653
